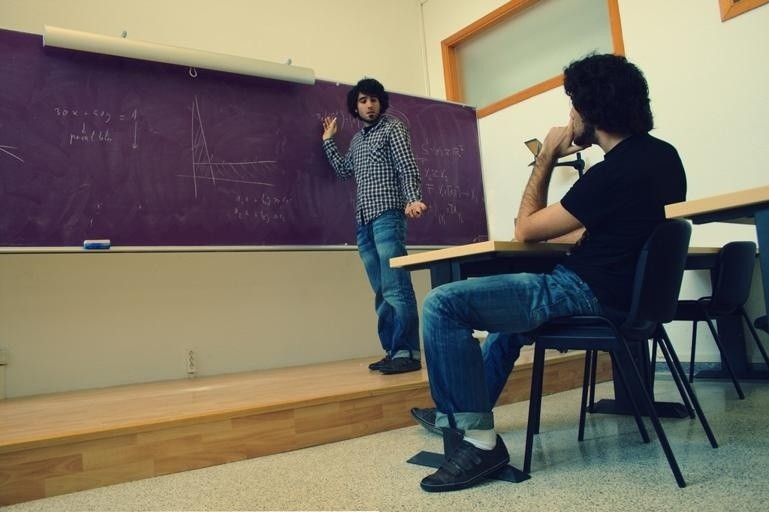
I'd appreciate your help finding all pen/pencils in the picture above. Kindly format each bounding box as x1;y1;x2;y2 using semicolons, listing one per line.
528;161;536;167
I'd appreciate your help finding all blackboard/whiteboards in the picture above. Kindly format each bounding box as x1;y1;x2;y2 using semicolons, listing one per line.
0;29;489;253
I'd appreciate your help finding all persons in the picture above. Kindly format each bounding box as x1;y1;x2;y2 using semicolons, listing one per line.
408;50;691;490
320;76;428;377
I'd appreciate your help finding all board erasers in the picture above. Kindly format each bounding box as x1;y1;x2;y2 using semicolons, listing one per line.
84;239;110;249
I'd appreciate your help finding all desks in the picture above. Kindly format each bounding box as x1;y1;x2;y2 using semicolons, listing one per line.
389;241;769;483
664;185;769;318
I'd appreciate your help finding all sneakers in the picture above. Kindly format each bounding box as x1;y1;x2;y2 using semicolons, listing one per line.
377;357;423;374
369;355;397;370
411;406;441;435
420;432;510;491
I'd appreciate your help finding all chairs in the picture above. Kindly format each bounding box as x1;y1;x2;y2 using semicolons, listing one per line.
648;241;769;400
520;218;720;490
